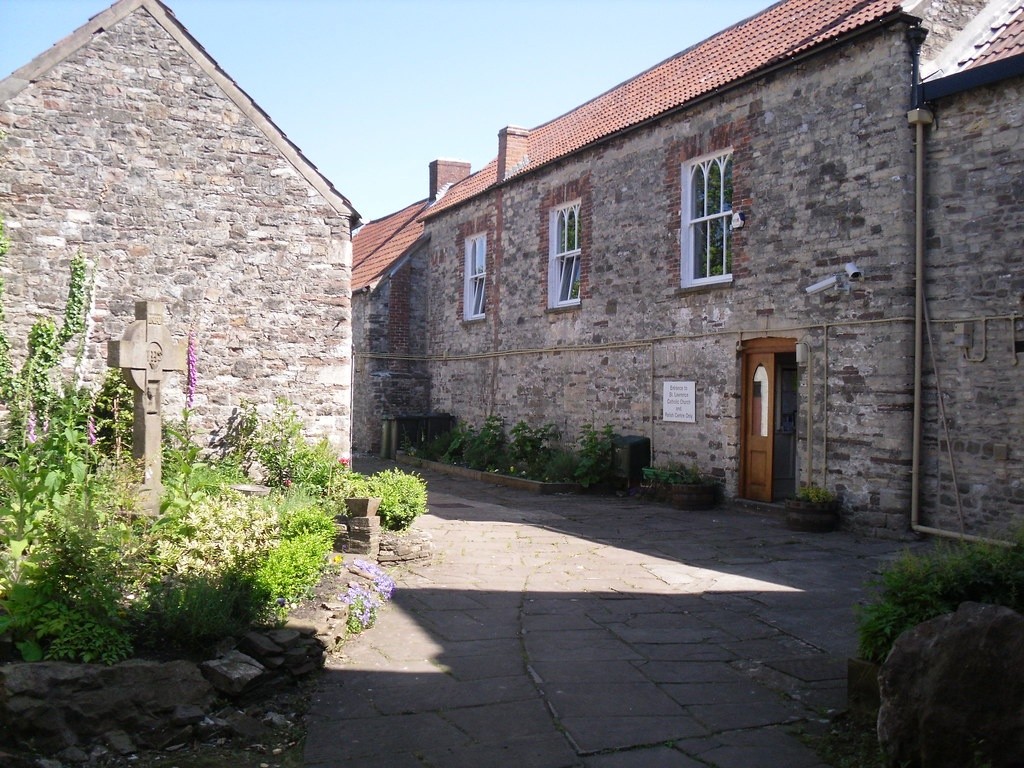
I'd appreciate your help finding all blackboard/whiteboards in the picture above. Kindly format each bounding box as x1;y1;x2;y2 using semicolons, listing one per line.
662;381;697;424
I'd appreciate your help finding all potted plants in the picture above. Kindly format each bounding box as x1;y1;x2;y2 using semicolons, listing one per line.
784;479;840;532
668;460;721;511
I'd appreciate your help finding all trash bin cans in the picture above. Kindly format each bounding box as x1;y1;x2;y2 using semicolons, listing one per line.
608;434;651;497
378;412;456;461
